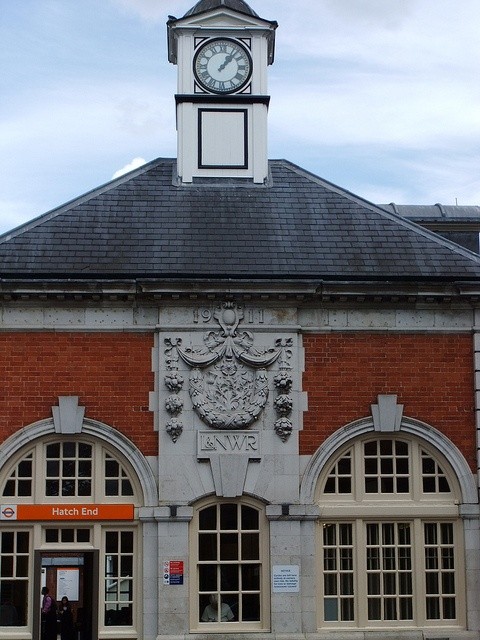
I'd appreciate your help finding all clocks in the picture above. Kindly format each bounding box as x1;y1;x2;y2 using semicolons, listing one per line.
193;37;253;95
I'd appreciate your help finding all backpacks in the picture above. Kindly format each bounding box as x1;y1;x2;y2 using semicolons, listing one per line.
44;596;58;613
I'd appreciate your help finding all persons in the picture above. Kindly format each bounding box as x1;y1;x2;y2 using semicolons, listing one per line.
41;586;58;640
58;596;72;636
201;594;235;622
